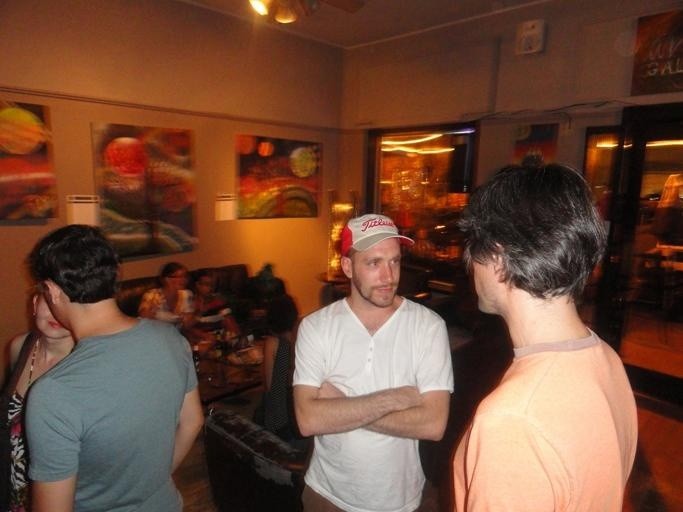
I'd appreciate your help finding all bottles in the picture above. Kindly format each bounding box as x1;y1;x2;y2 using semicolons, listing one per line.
214;330;235;358
192;345;200;374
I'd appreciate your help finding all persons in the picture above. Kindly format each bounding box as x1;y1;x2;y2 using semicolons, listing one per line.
447;152;642;511
0;286;76;511
193;273;254;350
21;223;207;511
137;262;198;334
289;212;456;511
251;295;299;438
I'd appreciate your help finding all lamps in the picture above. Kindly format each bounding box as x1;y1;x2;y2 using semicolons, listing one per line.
245;0;299;23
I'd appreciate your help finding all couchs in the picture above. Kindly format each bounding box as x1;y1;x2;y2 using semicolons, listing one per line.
112;263;515;510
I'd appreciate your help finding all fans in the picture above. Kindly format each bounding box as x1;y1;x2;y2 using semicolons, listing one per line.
295;0;369;21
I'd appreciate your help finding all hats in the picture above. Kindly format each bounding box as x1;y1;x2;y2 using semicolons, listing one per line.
338;212;416;259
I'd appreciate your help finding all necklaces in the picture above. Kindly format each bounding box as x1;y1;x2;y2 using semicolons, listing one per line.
27;339;41;397
